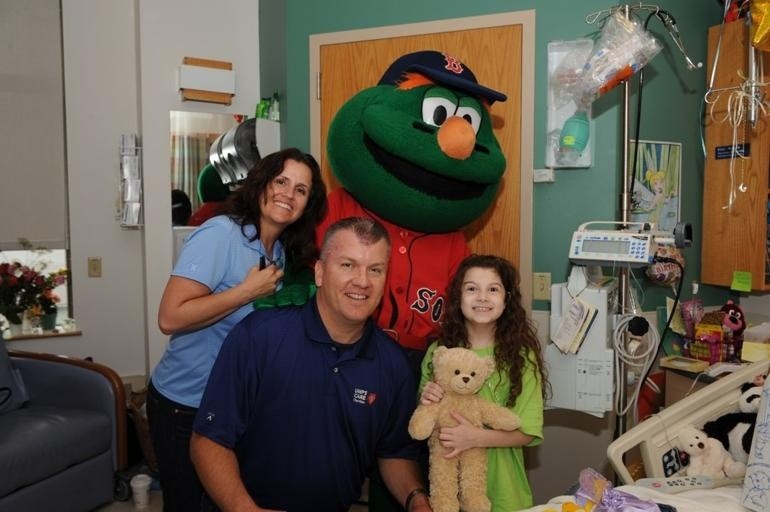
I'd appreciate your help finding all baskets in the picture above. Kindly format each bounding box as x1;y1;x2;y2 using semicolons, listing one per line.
680;335;731;366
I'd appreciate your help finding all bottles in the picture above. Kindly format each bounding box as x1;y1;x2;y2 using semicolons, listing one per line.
255;92;280;120
552;106;593;166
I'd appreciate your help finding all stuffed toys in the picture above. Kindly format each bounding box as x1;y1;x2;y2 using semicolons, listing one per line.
678;425;748;487
720;301;747;361
407;344;525;512
702;381;762;466
252;49;509;366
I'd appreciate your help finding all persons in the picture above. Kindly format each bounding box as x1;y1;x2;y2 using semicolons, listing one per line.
420;254;550;512
191;215;433;512
143;145;326;512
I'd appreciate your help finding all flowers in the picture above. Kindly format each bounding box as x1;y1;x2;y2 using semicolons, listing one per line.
37;269;68;314
0;261;51;323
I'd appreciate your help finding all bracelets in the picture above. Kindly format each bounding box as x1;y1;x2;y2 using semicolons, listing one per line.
403;489;432;512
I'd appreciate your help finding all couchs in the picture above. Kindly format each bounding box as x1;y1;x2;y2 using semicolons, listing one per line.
0;325;132;511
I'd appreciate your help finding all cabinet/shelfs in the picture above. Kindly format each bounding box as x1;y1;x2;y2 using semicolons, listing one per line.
697;16;770;293
658;359;730;409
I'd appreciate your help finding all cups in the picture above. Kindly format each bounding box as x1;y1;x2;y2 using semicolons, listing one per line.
130;474;154;510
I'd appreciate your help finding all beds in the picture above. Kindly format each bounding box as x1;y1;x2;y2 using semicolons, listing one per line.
515;348;770;512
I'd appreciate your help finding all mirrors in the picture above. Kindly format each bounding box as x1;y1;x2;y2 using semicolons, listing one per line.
166;107;250;231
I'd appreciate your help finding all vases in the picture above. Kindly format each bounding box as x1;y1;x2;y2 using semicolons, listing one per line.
6;307;24;339
38;306;58;329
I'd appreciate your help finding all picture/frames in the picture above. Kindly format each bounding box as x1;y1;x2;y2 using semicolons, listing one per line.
623;138;683;235
